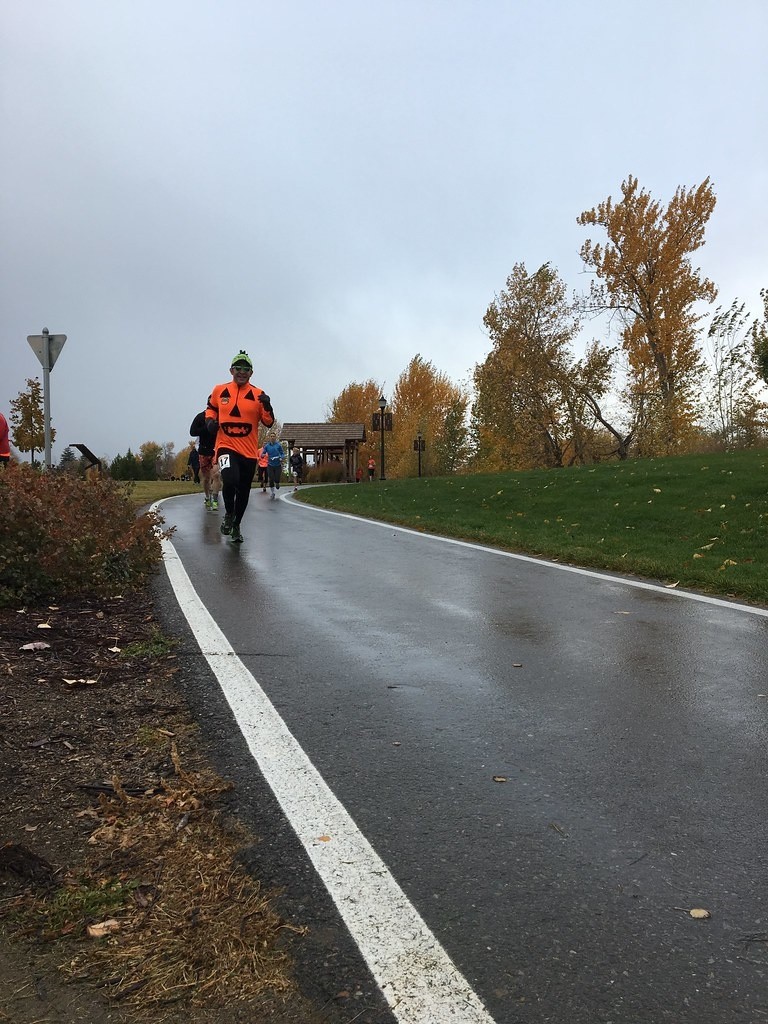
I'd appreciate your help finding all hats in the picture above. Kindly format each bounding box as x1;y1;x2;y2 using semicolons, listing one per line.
232;350;252;370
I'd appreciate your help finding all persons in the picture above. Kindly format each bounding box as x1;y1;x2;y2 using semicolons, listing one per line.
316;455;376;484
205;349;275;542
289;447;304;492
156;393;222;511
0;411;10;470
257;432;284;499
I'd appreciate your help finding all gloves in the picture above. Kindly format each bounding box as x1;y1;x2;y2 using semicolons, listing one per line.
206;417;220;432
258;391;270;412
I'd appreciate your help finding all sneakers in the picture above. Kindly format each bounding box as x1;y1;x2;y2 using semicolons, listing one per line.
212;499;219;511
230;531;244;542
220;513;236;535
203;498;212;511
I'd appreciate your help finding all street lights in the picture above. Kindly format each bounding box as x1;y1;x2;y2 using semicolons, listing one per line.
416;430;423;478
378;395;389;481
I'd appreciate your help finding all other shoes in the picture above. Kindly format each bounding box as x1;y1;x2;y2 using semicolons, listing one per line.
276;483;279;489
270;494;275;498
263;487;266;492
261;481;263;488
294;487;297;491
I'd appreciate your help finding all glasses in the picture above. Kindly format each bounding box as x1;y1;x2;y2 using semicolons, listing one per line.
232;365;252;373
